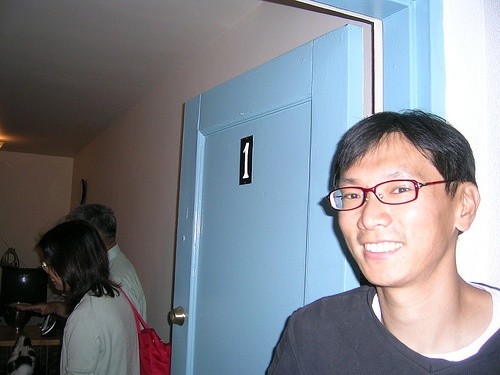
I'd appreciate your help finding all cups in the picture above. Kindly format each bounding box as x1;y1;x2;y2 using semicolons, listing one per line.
3;303;33;340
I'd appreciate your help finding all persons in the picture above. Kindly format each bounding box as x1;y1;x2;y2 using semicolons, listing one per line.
16;204;146;330
264;110;499;375
33;220;140;375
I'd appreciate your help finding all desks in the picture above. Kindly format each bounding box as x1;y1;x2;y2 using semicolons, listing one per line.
0;326;60;375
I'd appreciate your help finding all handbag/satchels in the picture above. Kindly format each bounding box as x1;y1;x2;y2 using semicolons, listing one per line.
113;281;171;375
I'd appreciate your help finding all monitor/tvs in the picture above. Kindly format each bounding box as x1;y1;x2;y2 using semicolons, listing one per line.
0;267;48;310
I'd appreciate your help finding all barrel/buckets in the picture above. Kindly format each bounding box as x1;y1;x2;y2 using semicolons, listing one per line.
1;266;47;319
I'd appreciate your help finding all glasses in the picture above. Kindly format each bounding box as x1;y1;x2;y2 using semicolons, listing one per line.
326;179;478;211
41;261;53;273
